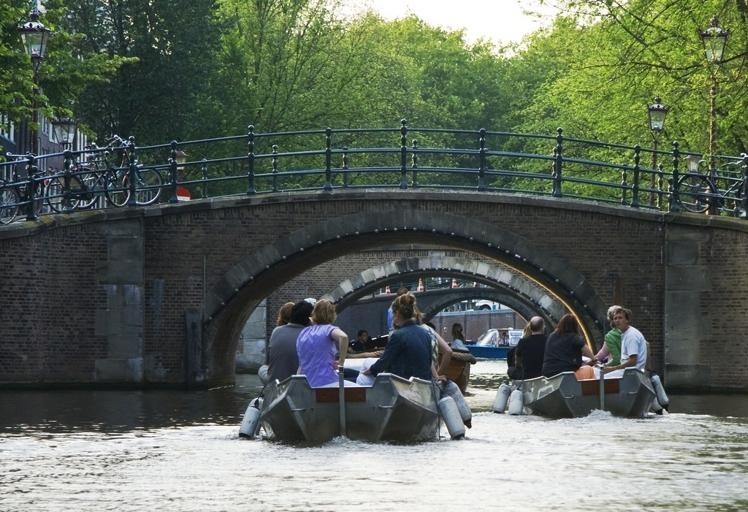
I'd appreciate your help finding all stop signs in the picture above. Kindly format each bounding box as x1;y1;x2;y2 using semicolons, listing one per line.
176;187;191;203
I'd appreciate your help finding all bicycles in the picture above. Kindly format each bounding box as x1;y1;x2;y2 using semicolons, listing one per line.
677;153;748;213
0;134;163;225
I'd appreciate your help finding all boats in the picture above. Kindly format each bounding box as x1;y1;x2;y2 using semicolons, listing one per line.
511;366;657;419
345;326;525;394
258;373;444;444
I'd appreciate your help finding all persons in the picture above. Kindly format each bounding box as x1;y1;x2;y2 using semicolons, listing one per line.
451;323;470;351
352;330;377;354
258;301;314;387
296;299;360;388
356;287;452;386
507;305;647;383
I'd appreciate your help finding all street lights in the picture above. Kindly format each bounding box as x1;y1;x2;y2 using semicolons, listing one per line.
697;16;730;214
173;148;187;188
16;6;51;220
51;115;78;212
646;95;702;212
